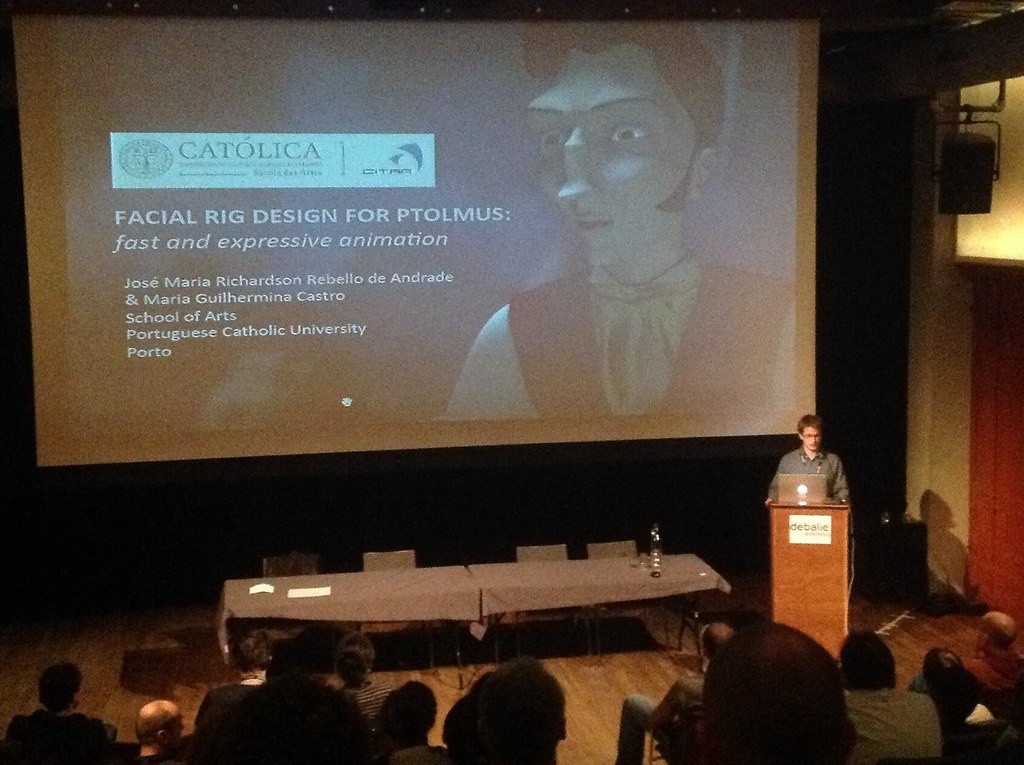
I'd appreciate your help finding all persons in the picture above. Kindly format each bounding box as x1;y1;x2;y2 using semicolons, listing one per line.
761;415;851;510
1;611;1024;765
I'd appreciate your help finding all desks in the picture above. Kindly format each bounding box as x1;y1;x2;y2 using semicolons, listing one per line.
867;522;928;599
467;553;724;668
213;565;481;690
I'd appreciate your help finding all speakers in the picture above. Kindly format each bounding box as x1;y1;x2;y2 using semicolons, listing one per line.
937;131;996;216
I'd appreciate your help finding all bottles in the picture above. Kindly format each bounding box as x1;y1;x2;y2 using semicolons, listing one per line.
648;524;663;570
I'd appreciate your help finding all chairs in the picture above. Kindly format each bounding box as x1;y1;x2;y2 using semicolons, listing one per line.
263;553;316;575
586;540;669;659
648;701;704;765
355;550;435;672
516;543;594;660
875;721;1023;765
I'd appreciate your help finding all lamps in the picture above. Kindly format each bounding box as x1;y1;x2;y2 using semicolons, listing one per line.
928;77;1006;215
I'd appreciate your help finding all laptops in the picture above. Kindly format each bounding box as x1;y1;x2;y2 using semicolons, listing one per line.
778;473;828;504
